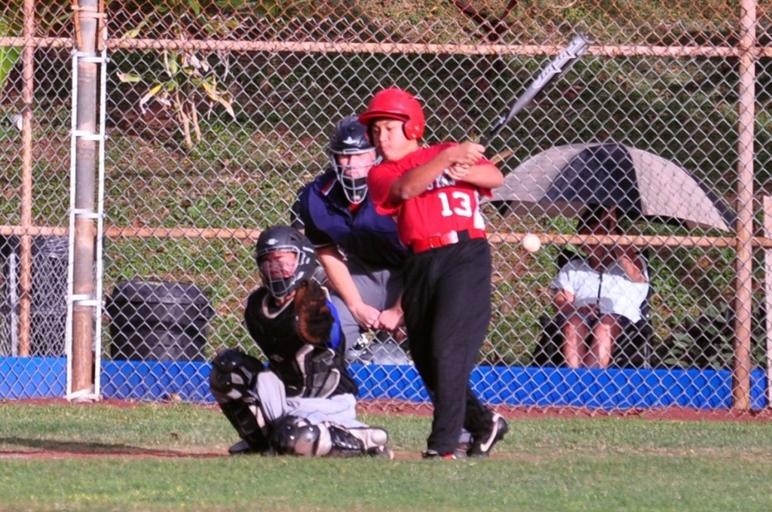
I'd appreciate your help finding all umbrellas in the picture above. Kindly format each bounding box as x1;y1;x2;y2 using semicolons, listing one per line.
478;140;730;306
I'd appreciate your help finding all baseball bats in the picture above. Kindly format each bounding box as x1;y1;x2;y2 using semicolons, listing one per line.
483;32;588;147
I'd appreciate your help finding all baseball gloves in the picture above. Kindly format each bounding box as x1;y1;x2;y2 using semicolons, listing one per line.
293;278;333;352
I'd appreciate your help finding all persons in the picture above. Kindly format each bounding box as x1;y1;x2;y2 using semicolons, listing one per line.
300;115;473;453
207;225;392;458
548;207;651;369
291;185;306;235
357;87;510;463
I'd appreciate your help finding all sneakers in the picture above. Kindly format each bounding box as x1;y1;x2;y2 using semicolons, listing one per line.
228;439;270;455
422;450;463;462
466;410;507;458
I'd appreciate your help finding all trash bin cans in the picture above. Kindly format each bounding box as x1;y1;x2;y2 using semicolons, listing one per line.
102;280;215;362
0;234;68;358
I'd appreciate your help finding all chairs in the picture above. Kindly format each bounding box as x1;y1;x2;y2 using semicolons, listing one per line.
531;248;655;368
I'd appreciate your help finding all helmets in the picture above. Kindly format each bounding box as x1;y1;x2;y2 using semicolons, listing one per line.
254;225;319;300
320;115;383;205
357;88;426;140
